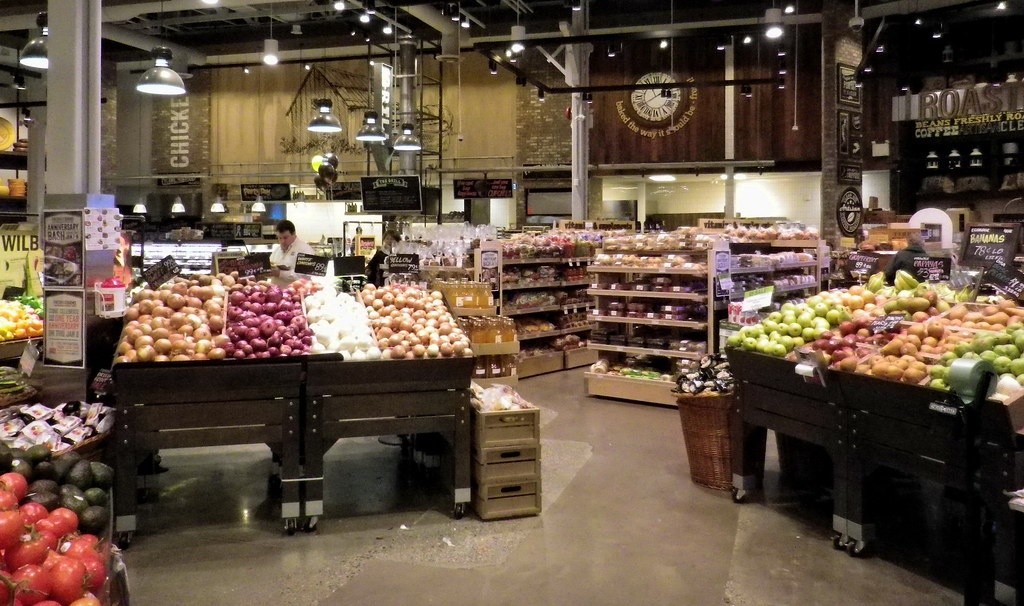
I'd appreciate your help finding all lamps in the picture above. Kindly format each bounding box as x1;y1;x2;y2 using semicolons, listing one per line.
13;74;25;89
488;57;544;102
356;33;387;142
306;0;343;133
263;0;278;66
19;1;51;70
135;0;186;95
582;87;593;104
22;106;31;121
740;81;753;97
661;85;673;98
607;39;617;58
132;49;308;213
776;42;787;89
393;78;422;151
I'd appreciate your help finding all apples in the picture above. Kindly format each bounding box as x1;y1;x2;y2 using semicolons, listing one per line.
727;286;884;357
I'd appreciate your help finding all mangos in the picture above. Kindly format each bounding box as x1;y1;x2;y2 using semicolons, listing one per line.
813;289;950;367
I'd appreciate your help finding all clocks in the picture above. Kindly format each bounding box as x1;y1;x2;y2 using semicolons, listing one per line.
631;72;681;122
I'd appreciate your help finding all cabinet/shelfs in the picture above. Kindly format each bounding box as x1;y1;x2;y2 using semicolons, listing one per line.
416;241;597;380
585;237;825;405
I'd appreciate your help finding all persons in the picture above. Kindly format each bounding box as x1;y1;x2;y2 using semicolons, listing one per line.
365;231;402;287
885;233;935;284
269;220;316;273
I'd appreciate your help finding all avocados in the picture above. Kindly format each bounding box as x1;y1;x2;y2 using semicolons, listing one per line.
0;439;114;534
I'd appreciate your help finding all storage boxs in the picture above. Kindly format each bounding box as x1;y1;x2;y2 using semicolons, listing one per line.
470;400;542;521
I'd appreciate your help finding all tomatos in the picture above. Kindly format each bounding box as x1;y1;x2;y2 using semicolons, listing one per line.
0;473;108;606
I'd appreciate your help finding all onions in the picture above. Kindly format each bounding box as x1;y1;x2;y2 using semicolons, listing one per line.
114;286;385;360
360;283;476;359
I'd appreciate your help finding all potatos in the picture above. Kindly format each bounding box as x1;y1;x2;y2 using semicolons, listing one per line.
171;271;320;293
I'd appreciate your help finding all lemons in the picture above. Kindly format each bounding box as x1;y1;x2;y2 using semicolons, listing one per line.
0;301;44;342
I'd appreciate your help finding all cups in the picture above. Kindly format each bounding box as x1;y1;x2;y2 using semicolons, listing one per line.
1004;142;1017;165
100;277;125;317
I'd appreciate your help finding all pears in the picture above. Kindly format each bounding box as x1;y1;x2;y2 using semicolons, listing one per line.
837;299;1024;392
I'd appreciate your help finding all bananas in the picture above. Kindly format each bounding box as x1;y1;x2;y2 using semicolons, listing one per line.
866;269;978;301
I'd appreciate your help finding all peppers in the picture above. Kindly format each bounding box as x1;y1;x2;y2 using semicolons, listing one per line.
15;296;44;318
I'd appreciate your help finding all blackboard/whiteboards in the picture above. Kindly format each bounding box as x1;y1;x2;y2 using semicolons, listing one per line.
361;176;422;213
846;253;879;274
907;256;951;282
326;182;362;200
975;258;1024;308
388;252;419;274
144;255;181;292
453;178;513;200
193;220;264;239
240;182;292;203
958;223;1019;266
870;314;905;329
295;252;327;277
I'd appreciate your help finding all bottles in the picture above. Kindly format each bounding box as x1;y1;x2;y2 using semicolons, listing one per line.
948;150;960;169
433;276;516;378
926;151;938;168
970;149;982;167
